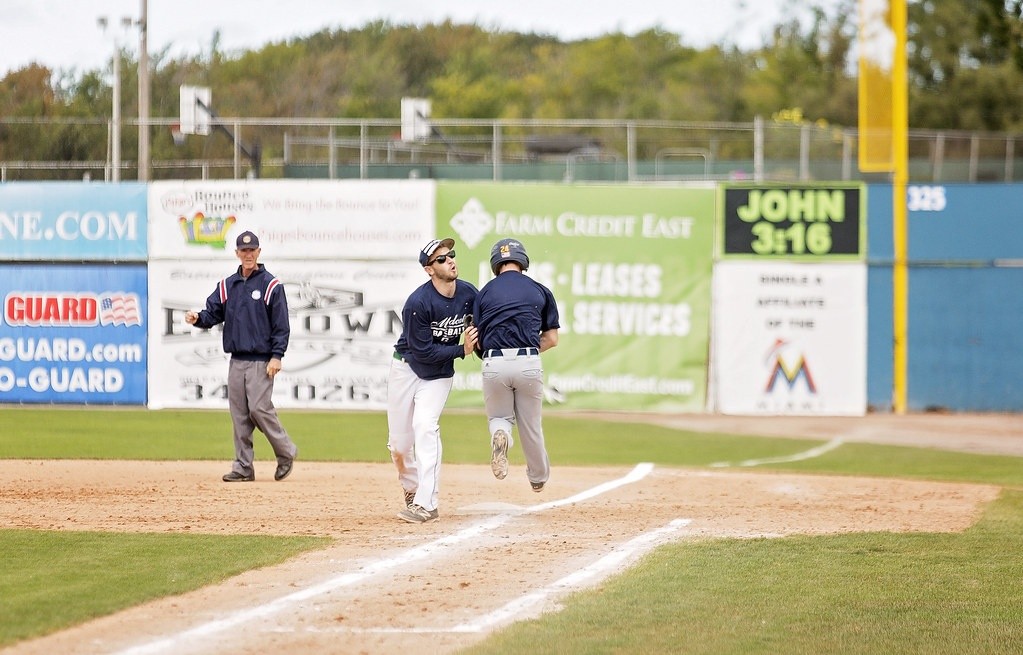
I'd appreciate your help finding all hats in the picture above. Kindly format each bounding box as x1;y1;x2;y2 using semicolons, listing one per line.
419;238;454;268
237;231;259;251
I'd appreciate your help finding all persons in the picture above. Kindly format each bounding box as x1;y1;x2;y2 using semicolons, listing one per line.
185;231;297;481
471;238;561;492
385;238;481;523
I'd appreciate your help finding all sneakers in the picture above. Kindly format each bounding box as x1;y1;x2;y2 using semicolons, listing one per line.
404;490;415;508
397;504;440;524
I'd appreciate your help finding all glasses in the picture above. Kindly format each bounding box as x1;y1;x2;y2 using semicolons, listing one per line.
428;251;456;267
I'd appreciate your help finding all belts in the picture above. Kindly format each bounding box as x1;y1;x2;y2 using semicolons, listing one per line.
481;347;539;358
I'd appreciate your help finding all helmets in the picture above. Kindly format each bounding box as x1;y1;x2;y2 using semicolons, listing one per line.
489;239;529;274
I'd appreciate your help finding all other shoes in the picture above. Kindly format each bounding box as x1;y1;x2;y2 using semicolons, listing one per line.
491;429;509;480
274;448;298;480
531;481;544;492
222;472;255;481
